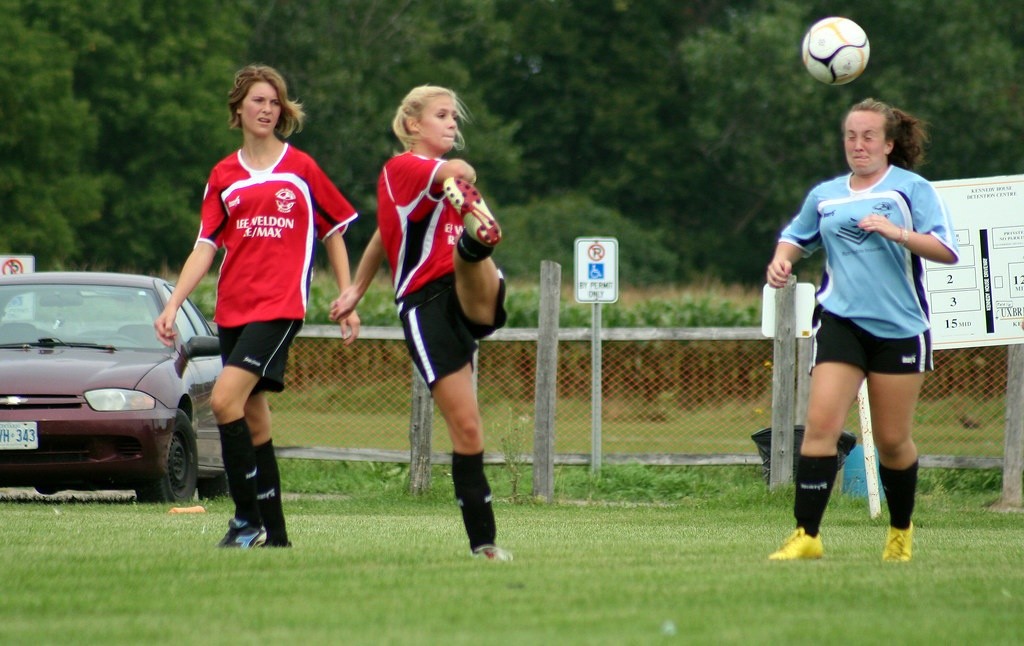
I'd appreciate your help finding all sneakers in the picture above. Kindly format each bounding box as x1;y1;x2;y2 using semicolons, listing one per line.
217;519;266;548
768;524;825;562
468;542;514;564
882;520;915;565
442;175;502;248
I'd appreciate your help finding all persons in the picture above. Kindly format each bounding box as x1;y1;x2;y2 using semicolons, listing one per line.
150;64;364;550
328;82;517;570
764;95;960;563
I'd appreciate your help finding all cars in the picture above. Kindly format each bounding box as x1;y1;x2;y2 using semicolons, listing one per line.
0;271;224;504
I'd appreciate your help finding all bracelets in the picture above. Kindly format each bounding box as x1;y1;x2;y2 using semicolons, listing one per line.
896;224;908;246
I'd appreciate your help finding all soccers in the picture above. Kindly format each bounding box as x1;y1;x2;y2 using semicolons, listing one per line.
800;16;872;86
